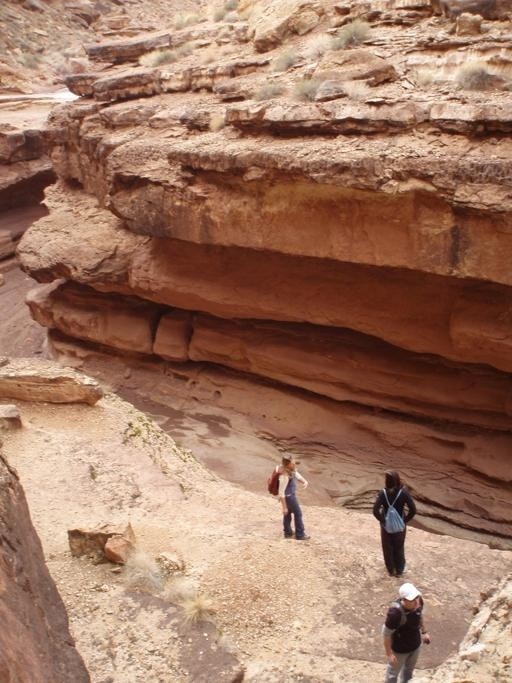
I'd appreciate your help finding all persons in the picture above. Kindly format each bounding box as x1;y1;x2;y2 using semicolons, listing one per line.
373;469;417;577
275;454;310;539
382;583;431;683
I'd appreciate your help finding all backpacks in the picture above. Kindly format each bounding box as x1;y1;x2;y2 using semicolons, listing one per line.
268;466;280;496
383;487;406;533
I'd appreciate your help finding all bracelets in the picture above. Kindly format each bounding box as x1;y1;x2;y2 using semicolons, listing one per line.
421;632;427;634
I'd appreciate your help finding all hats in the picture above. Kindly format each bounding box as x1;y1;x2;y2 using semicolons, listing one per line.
399;583;421;600
